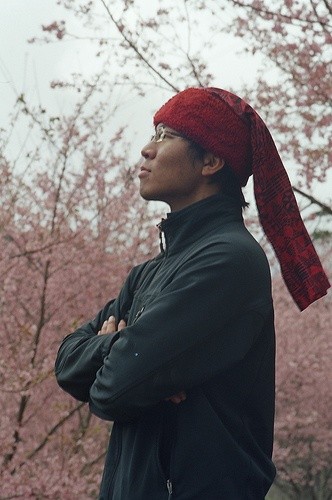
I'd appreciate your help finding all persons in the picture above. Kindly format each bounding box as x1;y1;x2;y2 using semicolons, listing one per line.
53;86;330;500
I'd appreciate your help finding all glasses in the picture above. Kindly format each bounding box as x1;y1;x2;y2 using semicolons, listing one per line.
150;126;184;143
